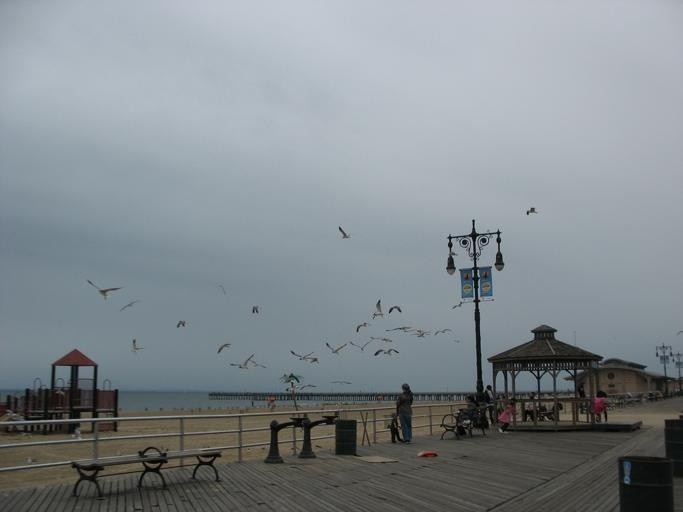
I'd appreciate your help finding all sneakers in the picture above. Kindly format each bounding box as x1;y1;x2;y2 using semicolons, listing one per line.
498;429;510;435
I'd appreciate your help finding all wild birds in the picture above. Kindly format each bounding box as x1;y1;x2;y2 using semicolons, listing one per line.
228;340;356;390
219;285;226;296
527;208;537;215
253;305;259;314
177;321;186;328
133;339;144;350
453;301;469;309
350;299;431;356
119;301;137;312
339;226;350;239
87;280;122;300
435;329;454;336
215;344;231;355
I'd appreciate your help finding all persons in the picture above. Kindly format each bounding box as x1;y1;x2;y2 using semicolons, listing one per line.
388;413;403;443
397;384;414;441
454;385;538;433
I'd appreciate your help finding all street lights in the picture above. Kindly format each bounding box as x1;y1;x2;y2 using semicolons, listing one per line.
444;219;509;402
653;340;682;400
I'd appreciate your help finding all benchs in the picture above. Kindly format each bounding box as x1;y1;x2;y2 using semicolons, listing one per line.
435;412;486;440
534;410;555;421
67;444;222;500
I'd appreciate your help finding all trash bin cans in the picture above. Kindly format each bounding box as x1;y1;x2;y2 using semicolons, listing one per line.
617;456;673;512
334;419;357;455
663;418;683;479
